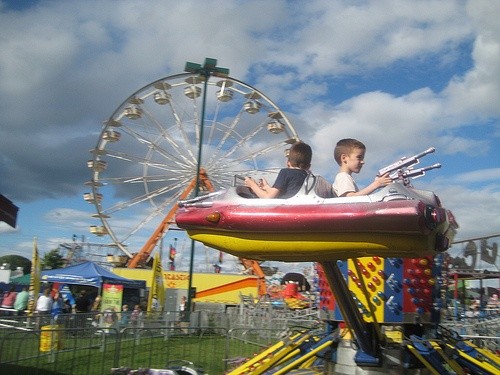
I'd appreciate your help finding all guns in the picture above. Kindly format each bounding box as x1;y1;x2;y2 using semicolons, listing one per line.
379;147;444;183
177;177;265;208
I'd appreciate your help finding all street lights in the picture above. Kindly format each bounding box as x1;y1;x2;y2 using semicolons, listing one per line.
182;58;229;324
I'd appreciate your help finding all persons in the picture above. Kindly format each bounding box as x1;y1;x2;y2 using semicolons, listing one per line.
119;305;131;323
73;290;90;337
14;287;29;310
1;286;17;309
244;143;312;199
51;293;63;317
331;139;392;197
179;296;188;322
132;305;143;315
36;289;53;314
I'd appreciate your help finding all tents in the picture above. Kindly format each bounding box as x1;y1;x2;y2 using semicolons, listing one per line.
11;260;146;297
